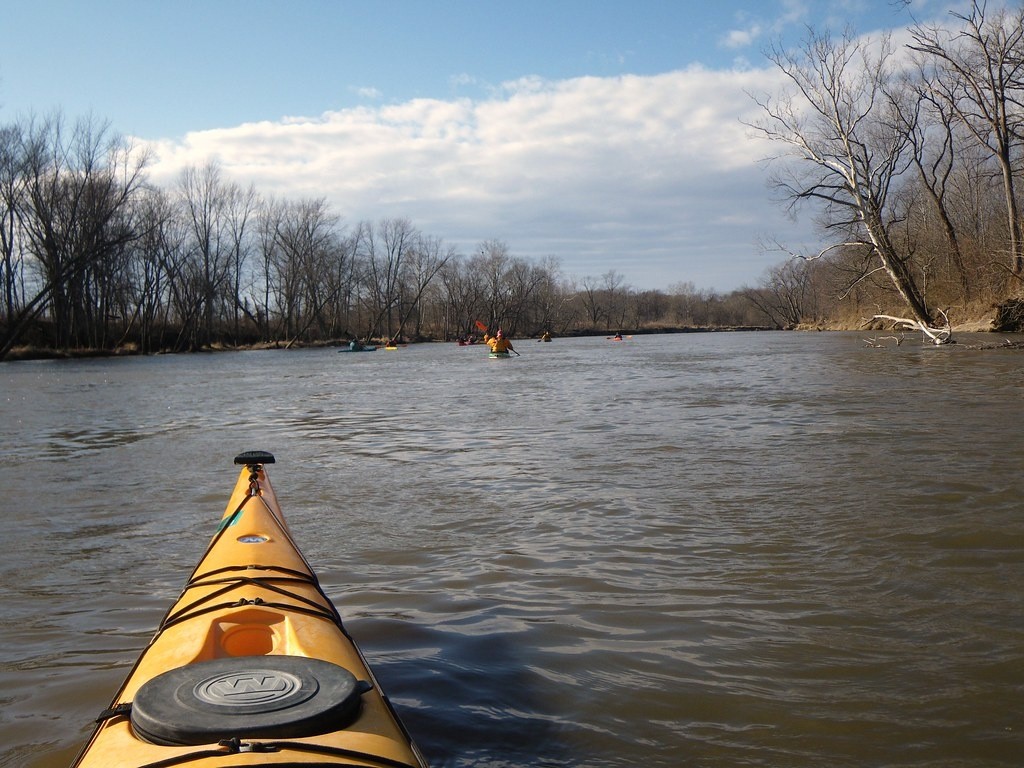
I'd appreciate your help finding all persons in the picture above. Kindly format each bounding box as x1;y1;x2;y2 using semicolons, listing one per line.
615;332;622;340
484;332;489;342
349;337;364;351
542;330;550;339
457;334;478;343
386;338;396;347
487;330;513;353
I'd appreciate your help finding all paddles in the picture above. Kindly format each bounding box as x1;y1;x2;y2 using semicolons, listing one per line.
537;337;547;342
376;344;407;349
474;320;520;356
607;335;632;339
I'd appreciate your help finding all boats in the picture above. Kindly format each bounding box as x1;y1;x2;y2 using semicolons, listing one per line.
457;341;486;345
80;450;424;761
614;337;621;340
544;338;552;341
490;353;512;358
384;347;399;350
338;347;377;351
